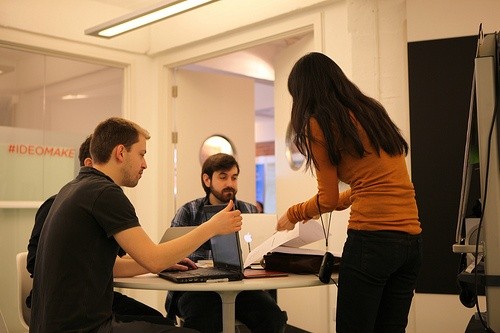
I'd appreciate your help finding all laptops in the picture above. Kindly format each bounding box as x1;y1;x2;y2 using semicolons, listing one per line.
158;224;197;245
161;202;245;282
198;212;279;264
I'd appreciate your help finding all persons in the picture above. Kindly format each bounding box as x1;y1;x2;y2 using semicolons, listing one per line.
170;153;288;333
25;135;198;332
277;52;424;333
28;117;243;332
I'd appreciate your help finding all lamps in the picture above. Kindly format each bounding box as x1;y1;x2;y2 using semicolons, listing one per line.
85;0;219;39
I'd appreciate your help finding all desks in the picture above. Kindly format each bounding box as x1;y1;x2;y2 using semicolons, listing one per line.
112;260;339;333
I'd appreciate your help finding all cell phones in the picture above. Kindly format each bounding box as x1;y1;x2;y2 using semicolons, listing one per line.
318;252;334;284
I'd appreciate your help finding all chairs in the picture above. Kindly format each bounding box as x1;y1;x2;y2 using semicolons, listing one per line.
16;251;33;329
